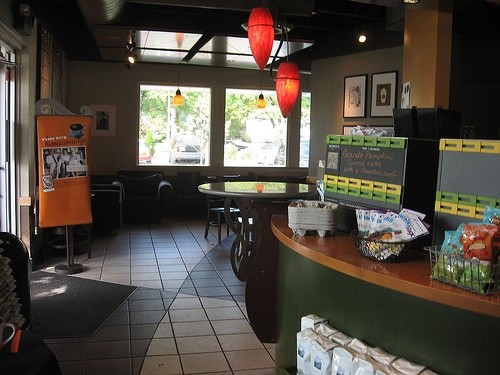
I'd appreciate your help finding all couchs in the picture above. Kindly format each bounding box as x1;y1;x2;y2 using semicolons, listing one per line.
117;169;176;237
90;174;125;238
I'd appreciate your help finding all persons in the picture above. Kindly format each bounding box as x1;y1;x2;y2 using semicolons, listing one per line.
45;148;84;179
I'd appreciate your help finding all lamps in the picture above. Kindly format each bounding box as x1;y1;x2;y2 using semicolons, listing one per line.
174;63;184;104
240;0;301;117
126;31;137;64
15;3;37;37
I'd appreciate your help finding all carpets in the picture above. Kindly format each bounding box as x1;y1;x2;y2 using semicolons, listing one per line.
31;270;140;338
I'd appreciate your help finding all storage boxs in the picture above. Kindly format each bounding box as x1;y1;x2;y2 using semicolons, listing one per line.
288;200;339;237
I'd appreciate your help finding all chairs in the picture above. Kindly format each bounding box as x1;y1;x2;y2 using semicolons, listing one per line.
0;230;34;354
176;171;242;244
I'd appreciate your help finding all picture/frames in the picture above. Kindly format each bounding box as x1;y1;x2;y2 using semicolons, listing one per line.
342;75;369;120
369;71;398;117
90;104;117;138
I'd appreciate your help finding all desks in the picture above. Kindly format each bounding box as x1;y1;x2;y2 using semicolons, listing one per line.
197;180;309;282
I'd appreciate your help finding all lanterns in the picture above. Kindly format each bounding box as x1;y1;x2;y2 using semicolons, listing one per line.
276;62;300;119
248;7;274;72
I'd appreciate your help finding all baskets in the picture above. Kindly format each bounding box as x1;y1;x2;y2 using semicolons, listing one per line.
349;229;409;262
423;245;500;296
